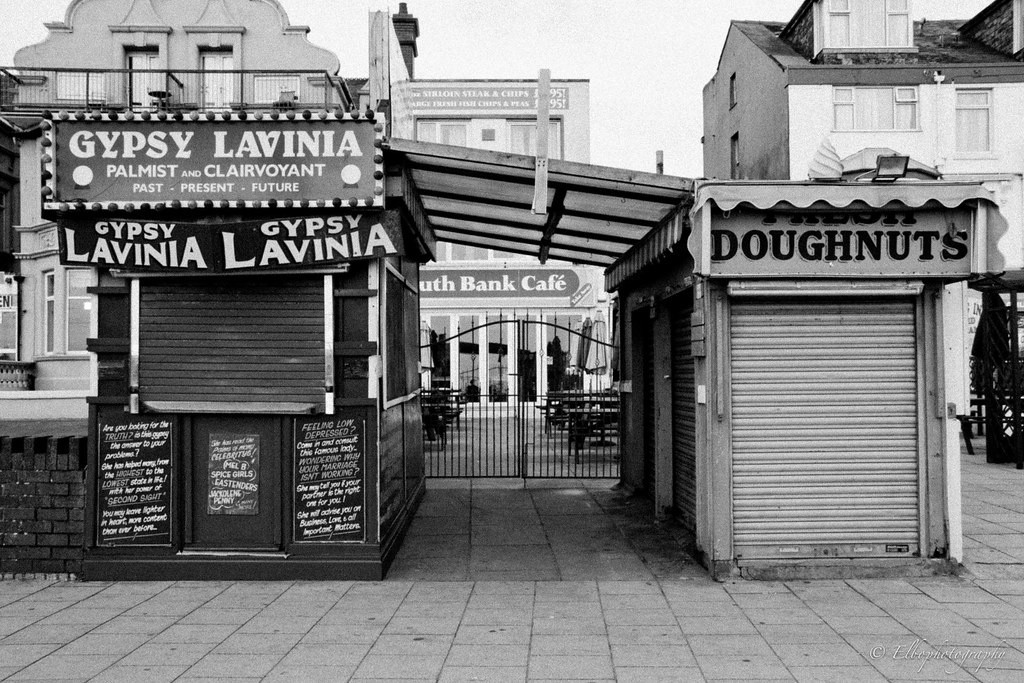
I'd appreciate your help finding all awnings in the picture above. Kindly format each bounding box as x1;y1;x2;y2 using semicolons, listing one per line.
577;309;608;377
420;321;434;372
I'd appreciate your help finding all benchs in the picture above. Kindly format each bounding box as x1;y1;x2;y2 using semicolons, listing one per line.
535;391;619;465
421;388;464;440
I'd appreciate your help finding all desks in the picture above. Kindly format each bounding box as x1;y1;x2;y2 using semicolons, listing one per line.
148;91;172;111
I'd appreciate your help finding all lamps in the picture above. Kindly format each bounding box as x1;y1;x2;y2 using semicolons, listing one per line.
871;153;909;183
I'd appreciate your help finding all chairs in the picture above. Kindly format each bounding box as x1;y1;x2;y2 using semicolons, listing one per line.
89;92;108;111
273;92;294;111
